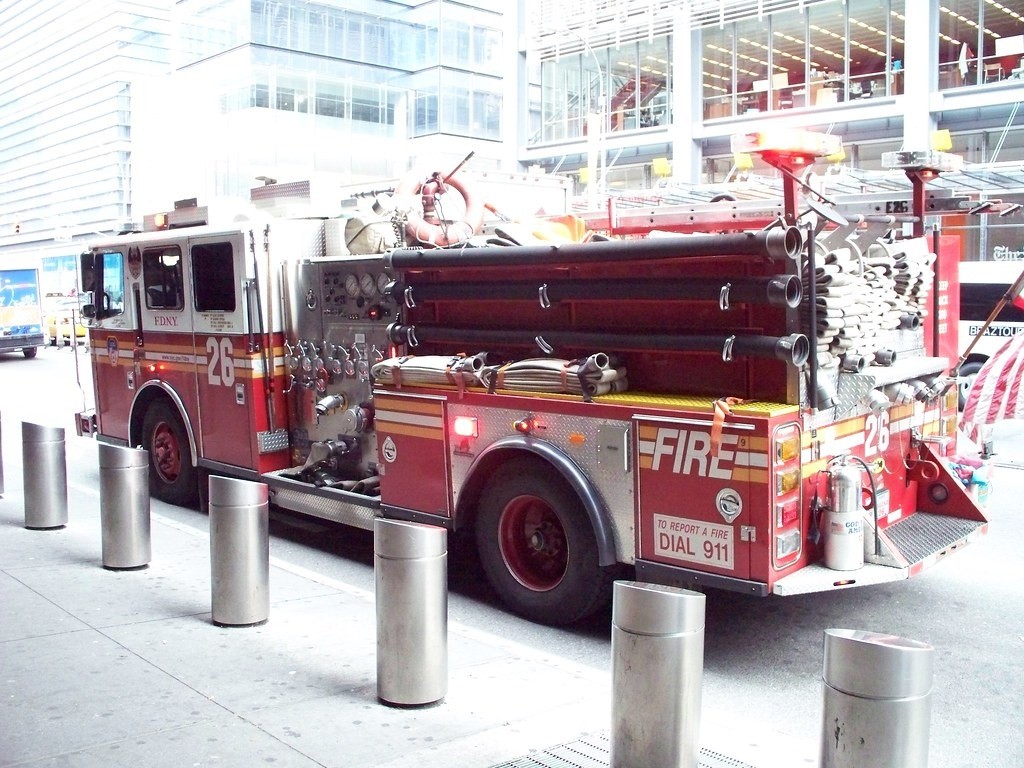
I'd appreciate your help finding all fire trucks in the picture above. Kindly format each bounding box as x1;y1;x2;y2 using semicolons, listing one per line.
77;132;997;628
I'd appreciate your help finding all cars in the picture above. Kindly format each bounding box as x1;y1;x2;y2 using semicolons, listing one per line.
43;297;88;340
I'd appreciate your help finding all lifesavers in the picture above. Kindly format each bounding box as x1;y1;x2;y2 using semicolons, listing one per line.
396;170;485;246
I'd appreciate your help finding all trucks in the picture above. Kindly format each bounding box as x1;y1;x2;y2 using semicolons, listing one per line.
0;268;46;358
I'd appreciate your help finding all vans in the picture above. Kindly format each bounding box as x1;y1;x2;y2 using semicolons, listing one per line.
958;257;1024;409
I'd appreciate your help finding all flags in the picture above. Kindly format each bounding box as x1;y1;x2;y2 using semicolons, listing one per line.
959;327;1024;452
